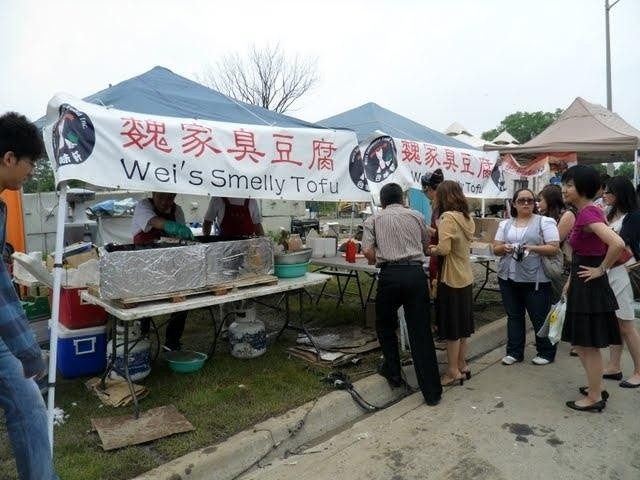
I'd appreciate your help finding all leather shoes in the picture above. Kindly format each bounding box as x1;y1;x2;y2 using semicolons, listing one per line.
620;377;640;388
570;347;578;357
602;371;624;381
376;366;402;388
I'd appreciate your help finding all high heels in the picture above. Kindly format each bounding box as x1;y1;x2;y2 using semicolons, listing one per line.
565;399;606;413
579;385;609;401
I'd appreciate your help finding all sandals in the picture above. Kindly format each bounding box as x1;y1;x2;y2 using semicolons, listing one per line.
440;371;465;387
460;365;471;379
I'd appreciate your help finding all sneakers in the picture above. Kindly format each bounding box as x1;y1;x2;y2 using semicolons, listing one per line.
501;355;517;366
531;355;552;365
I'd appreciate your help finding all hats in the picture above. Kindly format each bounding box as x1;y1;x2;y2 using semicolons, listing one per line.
417;167;444;190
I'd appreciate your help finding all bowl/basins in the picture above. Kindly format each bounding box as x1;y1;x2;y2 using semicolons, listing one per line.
274;248;314;278
164;351;208;373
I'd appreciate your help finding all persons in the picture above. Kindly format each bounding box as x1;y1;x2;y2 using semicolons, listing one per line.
202;196;264;338
1;112;57;479
362;183;442;406
421;172;444;291
491;166;640;412
425;181;475;386
131;191;194;353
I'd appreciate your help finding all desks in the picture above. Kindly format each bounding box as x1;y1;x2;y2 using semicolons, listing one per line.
311;255;501;328
78;272;334;420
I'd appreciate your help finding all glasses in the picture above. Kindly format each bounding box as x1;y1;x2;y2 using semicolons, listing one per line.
517;197;534;205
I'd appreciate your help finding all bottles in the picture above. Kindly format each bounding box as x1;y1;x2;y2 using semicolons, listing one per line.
346;238;356;263
288;232;303;253
83;224;92;243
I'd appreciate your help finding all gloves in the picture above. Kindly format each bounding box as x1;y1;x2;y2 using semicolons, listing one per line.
162;219;195;240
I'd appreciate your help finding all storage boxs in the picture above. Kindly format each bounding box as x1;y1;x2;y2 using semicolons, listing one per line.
469;241;495;255
46;246;100;272
473;217;500;242
47;286;107;328
48;318;106;380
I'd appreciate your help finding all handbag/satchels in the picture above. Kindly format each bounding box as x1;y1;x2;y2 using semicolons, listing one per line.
537;299;567;347
626;262;640;301
537;215;566;279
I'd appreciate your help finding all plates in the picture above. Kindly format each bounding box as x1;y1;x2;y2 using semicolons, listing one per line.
474;254;490;259
342;253;365;258
305;237;337;258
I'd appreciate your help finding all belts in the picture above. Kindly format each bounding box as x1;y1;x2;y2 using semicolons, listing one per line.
383;260;420;266
